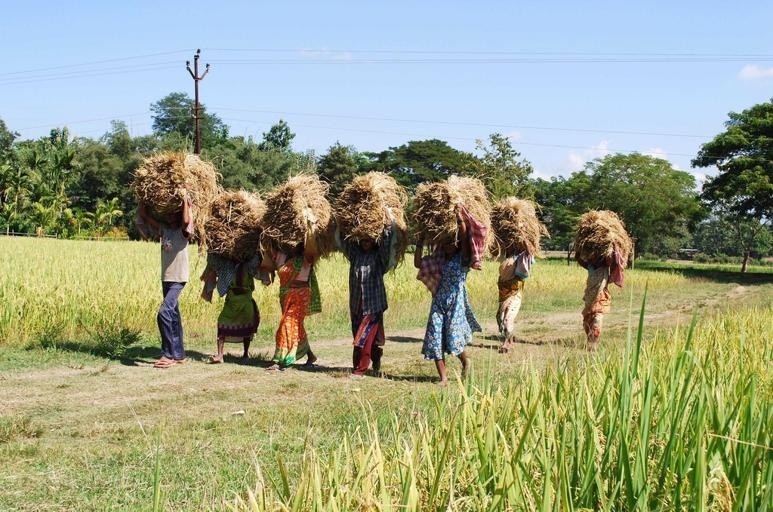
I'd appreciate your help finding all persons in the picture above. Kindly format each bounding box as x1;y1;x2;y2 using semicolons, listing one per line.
200;245;271;364
495;230;534;355
414;205;483;387
136;190;195;368
334;208;393;376
265;224;322;370
575;243;624;350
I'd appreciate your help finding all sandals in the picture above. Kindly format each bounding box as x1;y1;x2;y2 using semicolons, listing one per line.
173;358;188;364
153;358;175;368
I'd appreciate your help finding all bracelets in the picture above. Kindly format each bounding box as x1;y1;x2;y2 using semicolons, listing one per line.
457;219;464;223
416;245;423;249
